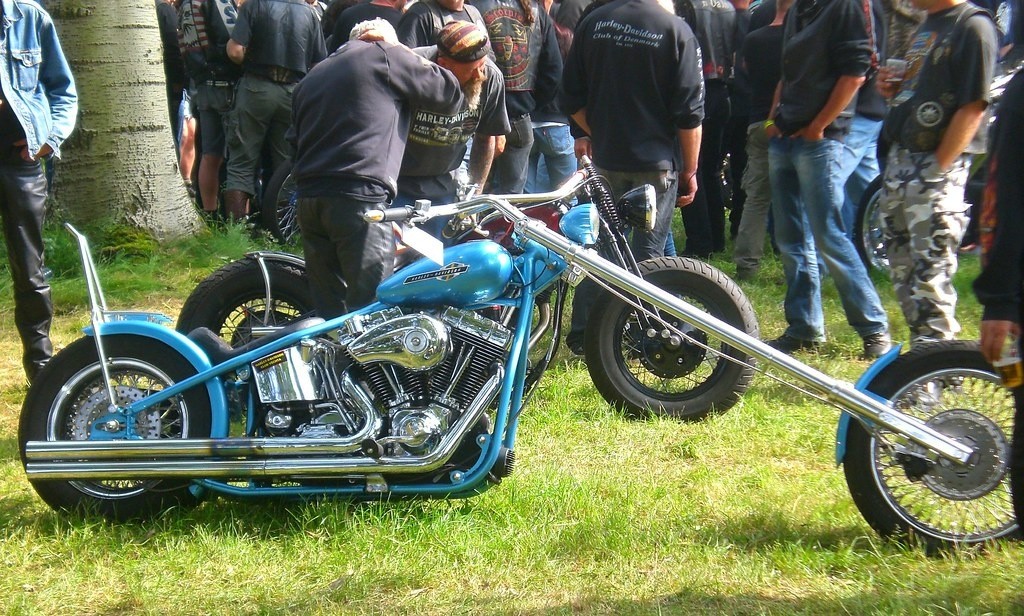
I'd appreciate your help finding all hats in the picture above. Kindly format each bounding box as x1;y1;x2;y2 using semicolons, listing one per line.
437;20;490;63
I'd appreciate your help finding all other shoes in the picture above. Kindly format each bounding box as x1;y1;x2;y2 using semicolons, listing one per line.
679;246;711;260
864;332;890;357
736;259;760;281
940;375;964;387
762;333;818;354
895;377;942;410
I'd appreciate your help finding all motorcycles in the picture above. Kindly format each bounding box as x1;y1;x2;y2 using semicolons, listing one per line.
17;139;1022;559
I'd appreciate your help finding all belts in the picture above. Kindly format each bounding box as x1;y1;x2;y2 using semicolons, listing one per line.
206;80;230;86
251;72;287;87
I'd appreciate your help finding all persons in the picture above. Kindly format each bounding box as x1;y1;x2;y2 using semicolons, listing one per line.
395;21;510;210
0;0;78;386
154;0;1023;541
294;16;464;310
